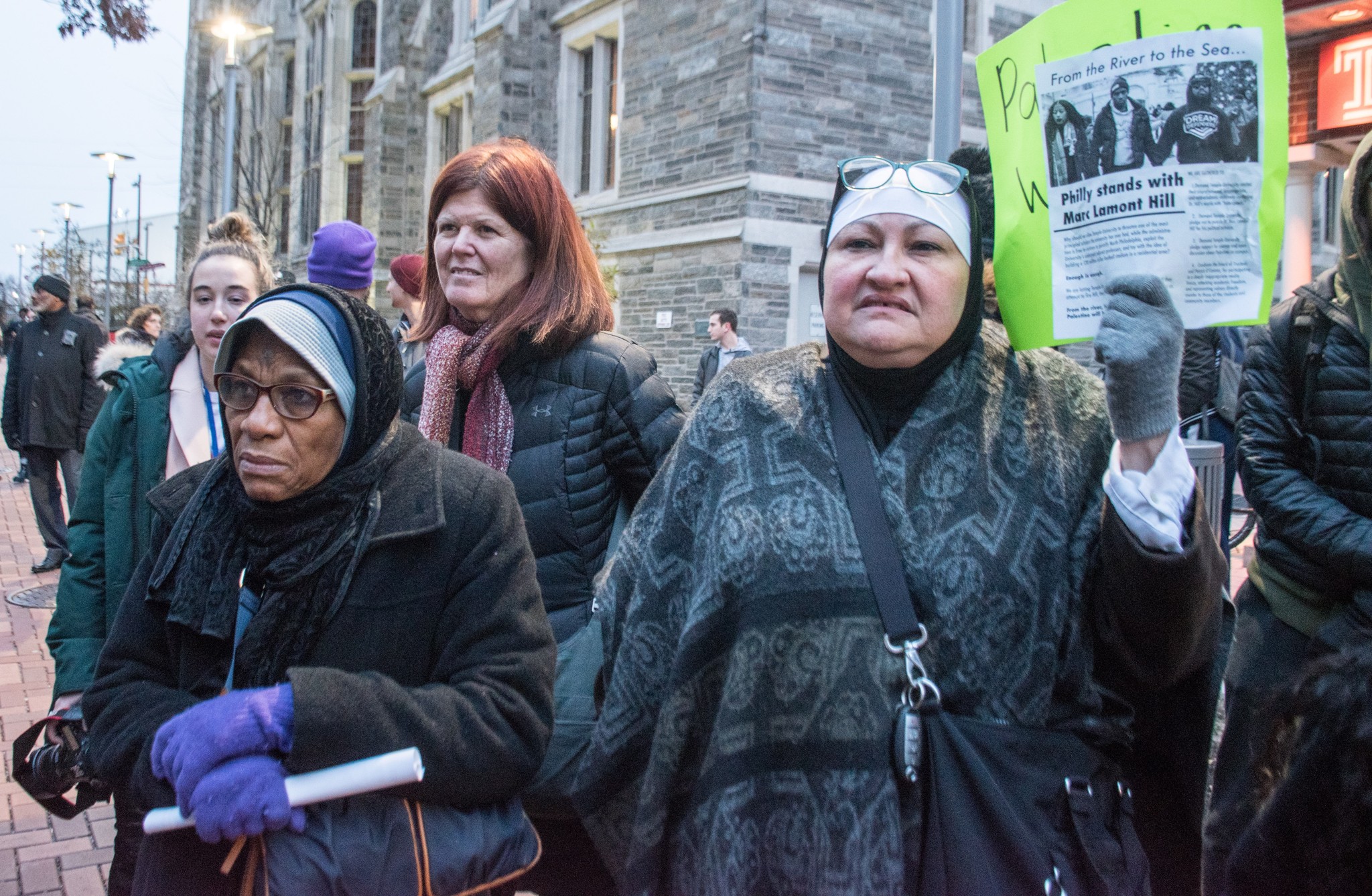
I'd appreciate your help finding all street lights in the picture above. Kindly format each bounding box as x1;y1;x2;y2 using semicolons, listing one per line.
15;243;27;300
53;201;84;278
32;228;55;276
92;150;136;329
209;13;274;220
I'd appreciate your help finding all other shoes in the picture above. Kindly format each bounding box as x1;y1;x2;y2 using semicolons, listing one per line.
13;469;30;482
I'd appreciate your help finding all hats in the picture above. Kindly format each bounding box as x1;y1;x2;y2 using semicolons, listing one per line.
826;164;972;268
34;273;71;303
1110;77;1128;97
213;297;357;421
248;289;359;387
391;255;428;300
307;220;377;289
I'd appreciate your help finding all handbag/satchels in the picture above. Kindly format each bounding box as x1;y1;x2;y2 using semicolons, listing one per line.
12;699;111;819
220;794;542;896
897;707;1155;896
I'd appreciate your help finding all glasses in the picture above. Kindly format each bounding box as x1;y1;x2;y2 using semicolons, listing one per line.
835;155;977;223
1192;82;1210;88
212;372;337;420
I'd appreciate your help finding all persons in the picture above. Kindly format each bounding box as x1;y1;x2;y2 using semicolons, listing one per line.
1199;129;1372;896
116;304;161;346
1042;73;1258;185
1217;647;1372;896
4;294;110;358
573;158;1229;896
397;137;687;896
948;148;1260;560
691;309;751;412
307;221;375;302
386;254;428;374
1;274;107;576
46;214;279;896
82;284;556;896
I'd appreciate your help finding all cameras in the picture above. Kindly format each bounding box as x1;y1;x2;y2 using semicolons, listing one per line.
25;701;113;800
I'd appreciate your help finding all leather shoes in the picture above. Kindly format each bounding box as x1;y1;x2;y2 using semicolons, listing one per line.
31;558;62;572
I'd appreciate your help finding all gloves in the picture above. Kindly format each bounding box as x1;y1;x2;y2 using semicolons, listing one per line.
187;754;303;843
150;683;300;819
1094;273;1185;442
